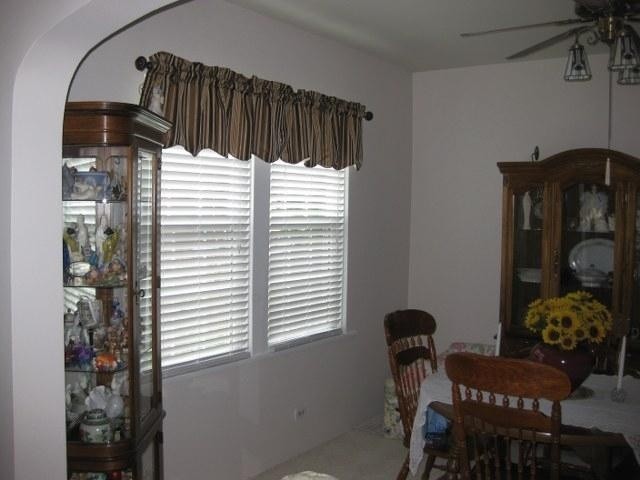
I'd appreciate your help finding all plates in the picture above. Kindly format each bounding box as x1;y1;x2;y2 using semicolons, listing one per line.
567;238;615;273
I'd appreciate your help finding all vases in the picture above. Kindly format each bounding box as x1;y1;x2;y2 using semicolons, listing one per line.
529;343;594;394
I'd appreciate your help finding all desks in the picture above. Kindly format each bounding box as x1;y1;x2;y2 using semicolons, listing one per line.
409;366;640;480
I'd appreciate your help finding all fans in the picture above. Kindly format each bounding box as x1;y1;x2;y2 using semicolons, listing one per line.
461;0;640;60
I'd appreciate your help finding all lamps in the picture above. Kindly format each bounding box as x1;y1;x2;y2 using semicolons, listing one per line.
564;27;640;85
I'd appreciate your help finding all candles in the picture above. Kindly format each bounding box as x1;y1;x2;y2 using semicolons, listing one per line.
617;335;626;389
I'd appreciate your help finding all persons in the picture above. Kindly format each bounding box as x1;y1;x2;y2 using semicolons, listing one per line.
77;214;88;246
95;215;108;251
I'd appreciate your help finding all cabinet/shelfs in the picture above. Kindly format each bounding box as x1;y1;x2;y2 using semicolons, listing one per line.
496;148;640;379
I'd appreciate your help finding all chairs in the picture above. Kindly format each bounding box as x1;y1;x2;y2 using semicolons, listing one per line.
384;309;494;480
445;353;571;480
